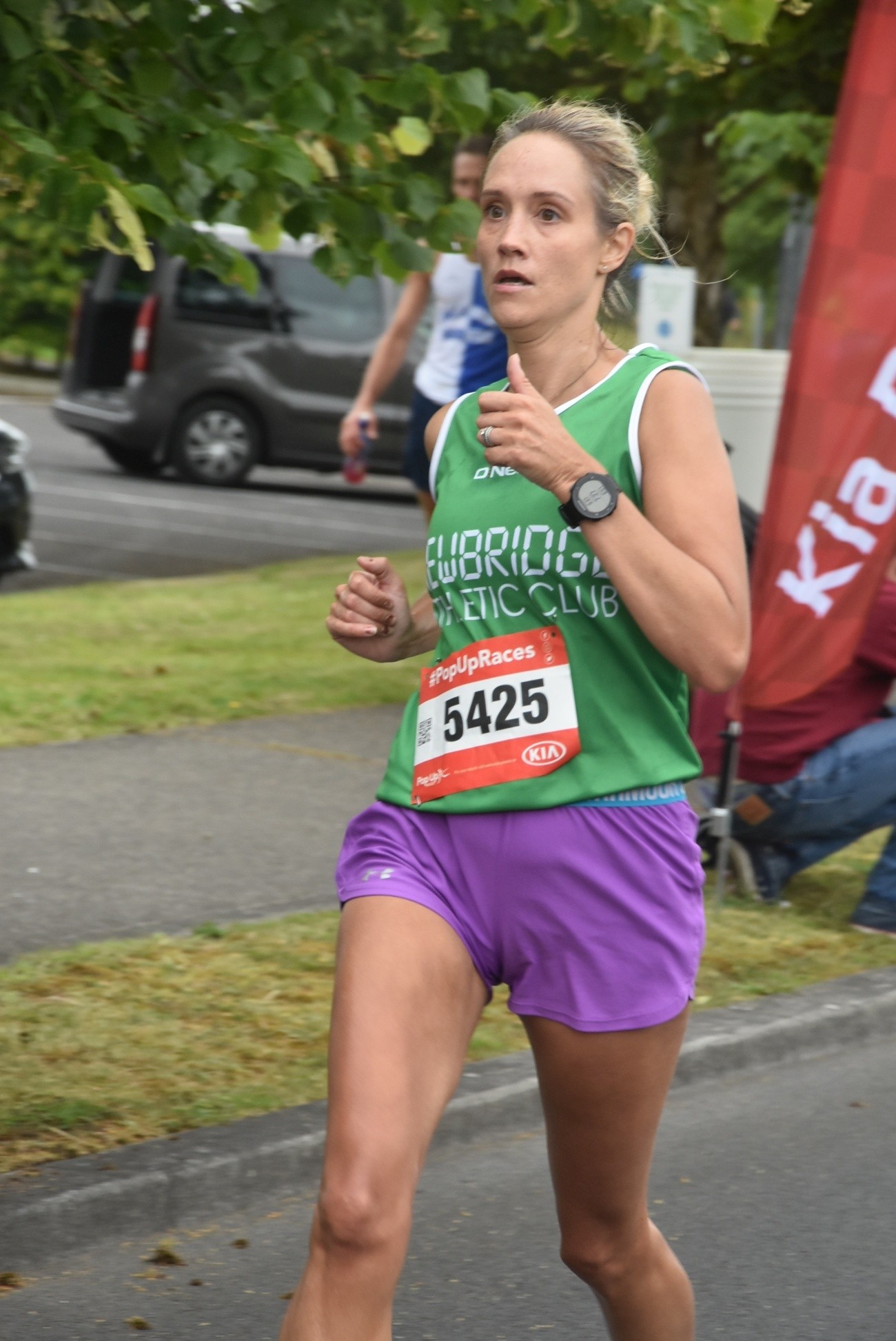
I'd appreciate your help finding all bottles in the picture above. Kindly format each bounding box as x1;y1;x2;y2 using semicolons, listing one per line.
342;419;371;484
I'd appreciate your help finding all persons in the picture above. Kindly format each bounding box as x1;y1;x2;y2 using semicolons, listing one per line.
698;712;896;946
285;100;752;1341
340;134;510;539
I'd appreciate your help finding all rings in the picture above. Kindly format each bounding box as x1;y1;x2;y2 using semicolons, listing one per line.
477;426;495;448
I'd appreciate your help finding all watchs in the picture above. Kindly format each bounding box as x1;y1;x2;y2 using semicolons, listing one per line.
557;469;623;529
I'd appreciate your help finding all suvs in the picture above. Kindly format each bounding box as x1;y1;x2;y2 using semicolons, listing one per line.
51;217;437;491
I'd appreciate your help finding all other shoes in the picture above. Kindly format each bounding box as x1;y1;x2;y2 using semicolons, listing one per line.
728;836;777;902
847;891;895;935
